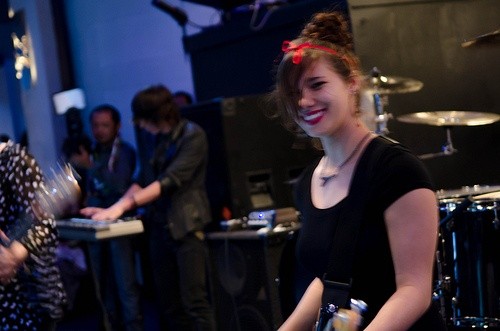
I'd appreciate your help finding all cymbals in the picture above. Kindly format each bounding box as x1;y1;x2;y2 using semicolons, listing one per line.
357;74;424;94
463;29;500;48
396;110;500;126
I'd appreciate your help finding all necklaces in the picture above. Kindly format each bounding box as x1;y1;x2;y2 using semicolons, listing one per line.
318;131;371;187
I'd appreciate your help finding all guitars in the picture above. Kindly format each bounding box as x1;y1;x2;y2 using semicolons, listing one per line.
311;297;367;331
0;154;82;247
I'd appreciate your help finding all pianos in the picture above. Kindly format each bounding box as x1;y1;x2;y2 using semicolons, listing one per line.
55;215;145;242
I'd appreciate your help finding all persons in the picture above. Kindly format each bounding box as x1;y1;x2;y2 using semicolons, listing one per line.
273;12;447;331
0;85;219;331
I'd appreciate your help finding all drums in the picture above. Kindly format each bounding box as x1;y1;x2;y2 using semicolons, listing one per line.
434;183;500;329
